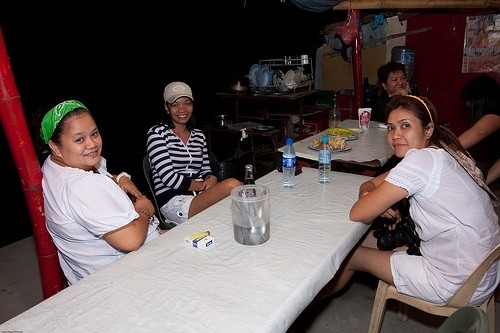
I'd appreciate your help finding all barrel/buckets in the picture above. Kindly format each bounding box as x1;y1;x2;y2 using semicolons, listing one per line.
230;184;271;245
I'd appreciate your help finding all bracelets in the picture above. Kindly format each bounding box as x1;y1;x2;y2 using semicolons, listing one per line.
137;210;150;217
205;174;216;181
116;172;131;184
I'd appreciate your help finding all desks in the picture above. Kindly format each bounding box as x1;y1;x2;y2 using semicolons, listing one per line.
215;90;317;139
0;121;399;333
204;126;281;174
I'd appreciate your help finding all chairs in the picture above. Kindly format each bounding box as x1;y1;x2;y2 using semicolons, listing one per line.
144;156;176;228
369;244;500;333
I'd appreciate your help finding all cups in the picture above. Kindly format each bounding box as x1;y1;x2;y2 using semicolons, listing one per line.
301;55;310;65
284;55;292;65
292;55;298;64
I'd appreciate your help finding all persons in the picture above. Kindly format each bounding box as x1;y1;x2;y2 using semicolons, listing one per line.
146;82;244;225
366;62;413;124
449;102;500;187
312;96;500;307
36;97;164;286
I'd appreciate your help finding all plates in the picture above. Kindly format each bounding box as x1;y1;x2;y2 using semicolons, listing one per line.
249;63;284;94
307;142;351;153
320;128;365;141
284;70;298;89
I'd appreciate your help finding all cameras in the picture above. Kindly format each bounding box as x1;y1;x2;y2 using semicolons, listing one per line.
375;217;408;251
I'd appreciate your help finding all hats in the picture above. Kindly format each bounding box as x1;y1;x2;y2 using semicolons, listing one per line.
163;82;194;105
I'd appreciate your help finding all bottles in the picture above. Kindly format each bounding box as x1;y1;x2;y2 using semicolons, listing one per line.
391;46;415;81
363;77;376;108
282;139;297;189
239;164;257;198
328;99;342;129
318;137;332;183
410;83;419;97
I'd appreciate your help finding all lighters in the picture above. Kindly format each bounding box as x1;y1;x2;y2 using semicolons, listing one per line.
189;230;211;240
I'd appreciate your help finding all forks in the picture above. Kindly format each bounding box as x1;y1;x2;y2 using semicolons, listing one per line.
241;225;252;245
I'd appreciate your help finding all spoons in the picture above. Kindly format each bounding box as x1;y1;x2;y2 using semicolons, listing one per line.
235;228;244;244
255;190;267;234
240;203;261;244
